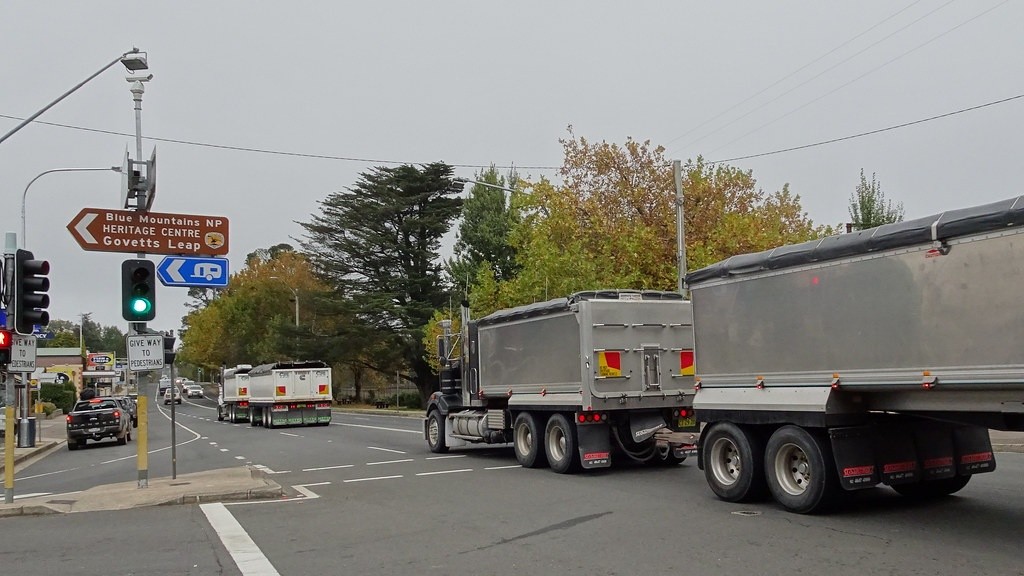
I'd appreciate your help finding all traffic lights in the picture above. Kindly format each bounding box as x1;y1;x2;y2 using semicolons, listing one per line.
122;259;156;323
0;331;12;366
12;249;51;337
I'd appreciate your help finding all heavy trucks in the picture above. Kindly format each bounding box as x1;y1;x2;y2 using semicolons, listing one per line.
430;190;1024;515
218;360;334;426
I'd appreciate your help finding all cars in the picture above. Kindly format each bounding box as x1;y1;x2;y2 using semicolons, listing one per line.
115;394;138;428
187;385;204;398
164;388;182;405
159;374;196;396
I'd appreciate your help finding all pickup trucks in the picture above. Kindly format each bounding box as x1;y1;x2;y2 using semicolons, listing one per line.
65;398;132;452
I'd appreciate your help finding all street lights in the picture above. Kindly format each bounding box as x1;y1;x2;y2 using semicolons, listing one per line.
269;275;302;354
451;178;551;222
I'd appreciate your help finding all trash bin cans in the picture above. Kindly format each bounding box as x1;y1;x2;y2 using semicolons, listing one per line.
18;417;37;449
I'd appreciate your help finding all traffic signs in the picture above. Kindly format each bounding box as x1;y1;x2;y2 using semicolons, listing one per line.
67;208;230;258
